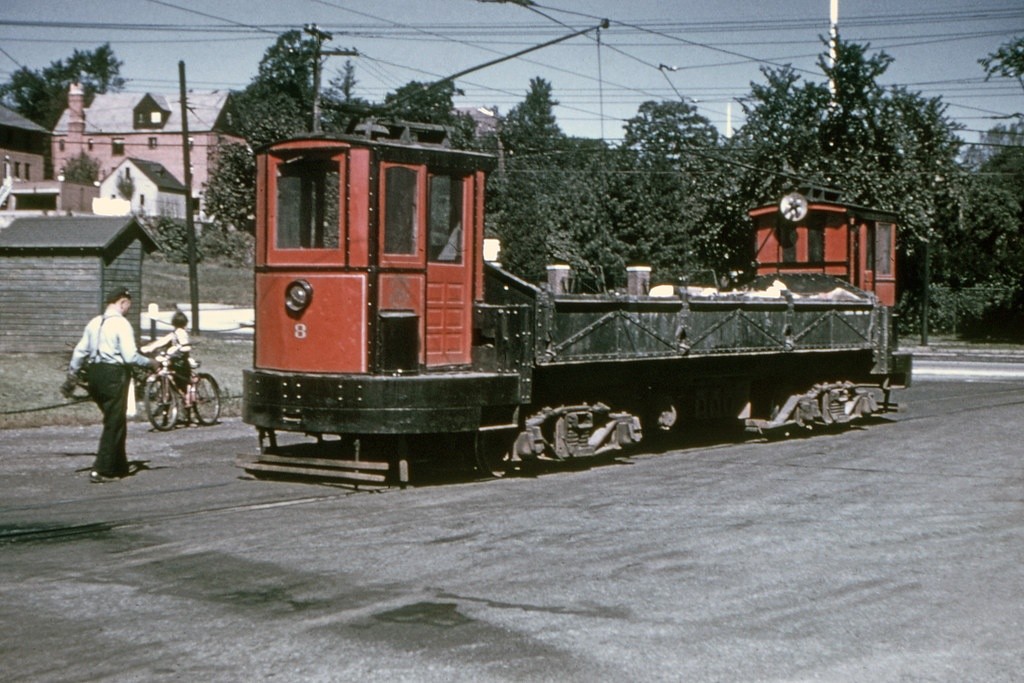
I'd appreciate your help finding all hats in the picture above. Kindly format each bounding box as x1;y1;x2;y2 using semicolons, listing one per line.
104;286;132;302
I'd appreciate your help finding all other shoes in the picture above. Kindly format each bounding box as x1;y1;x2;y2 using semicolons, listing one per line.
89;470;121;482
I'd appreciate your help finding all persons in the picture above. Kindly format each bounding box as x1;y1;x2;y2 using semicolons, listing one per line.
68;287;160;484
141;312;196;406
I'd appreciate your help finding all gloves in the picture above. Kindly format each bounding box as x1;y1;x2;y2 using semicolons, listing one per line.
60;373;79;399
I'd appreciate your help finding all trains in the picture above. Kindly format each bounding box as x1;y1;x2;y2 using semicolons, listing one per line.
237;114;912;494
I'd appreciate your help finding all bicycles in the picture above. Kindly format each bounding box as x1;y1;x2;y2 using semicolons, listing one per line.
144;345;223;432
